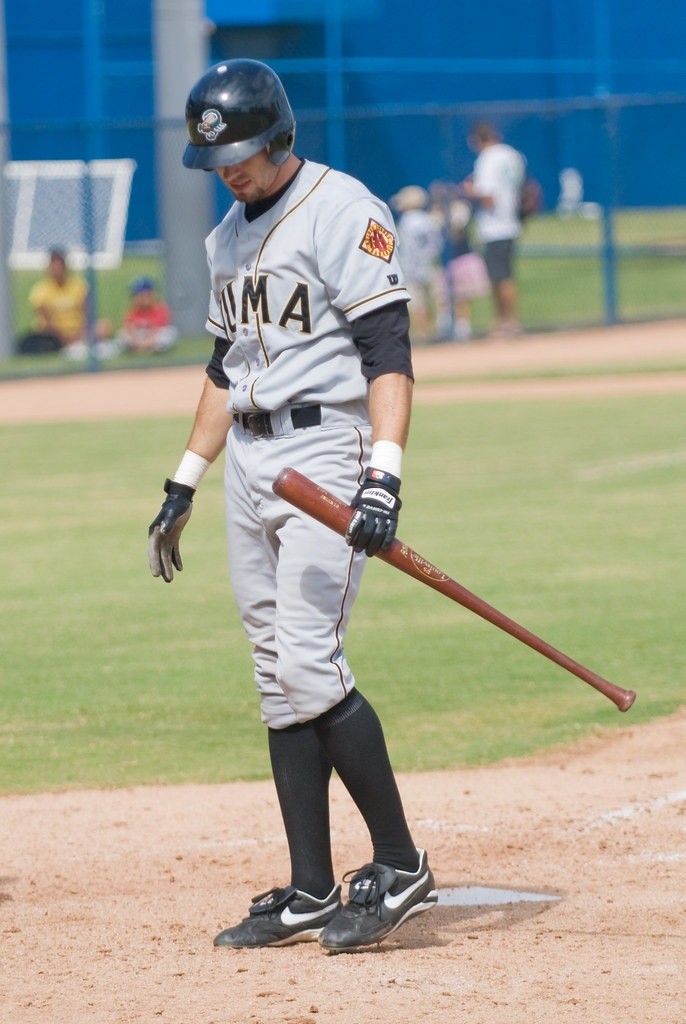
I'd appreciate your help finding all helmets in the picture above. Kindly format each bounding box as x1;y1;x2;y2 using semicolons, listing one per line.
182;58;296;169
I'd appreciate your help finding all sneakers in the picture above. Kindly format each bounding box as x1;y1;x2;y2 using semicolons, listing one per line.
318;848;438;955
214;884;344;948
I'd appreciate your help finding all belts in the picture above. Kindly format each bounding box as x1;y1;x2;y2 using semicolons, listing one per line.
233;405;321;438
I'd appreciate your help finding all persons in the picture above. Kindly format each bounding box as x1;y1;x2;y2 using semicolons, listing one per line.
388;119;538;351
147;57;440;954
13;247;177;364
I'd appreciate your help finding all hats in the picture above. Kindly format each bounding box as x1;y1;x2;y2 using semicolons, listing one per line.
130;276;152;294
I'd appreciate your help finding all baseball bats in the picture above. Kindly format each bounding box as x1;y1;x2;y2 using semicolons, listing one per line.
269;463;638;711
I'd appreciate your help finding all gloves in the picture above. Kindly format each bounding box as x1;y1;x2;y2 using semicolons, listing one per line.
148;478;196;583
345;466;402;558
